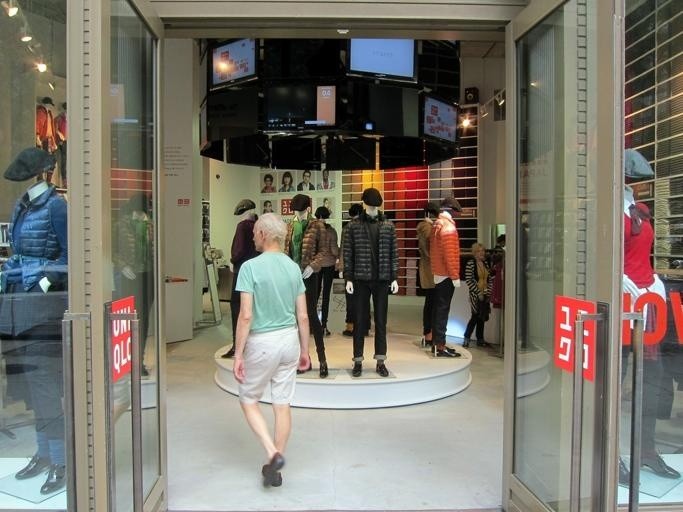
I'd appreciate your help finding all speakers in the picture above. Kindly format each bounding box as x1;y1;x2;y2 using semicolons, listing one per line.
465;87;479;104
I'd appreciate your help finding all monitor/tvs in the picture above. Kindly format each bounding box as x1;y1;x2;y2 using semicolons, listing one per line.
346;38;420;85
200;98;211;152
226;130;271;167
262;38;345;81
379;136;425;169
425;143;459;166
270;135;323;171
418;90;458;146
325;136;377;171
200;141;225;163
337;77;421;140
256;79;337;129
206;38;259;92
206;80;258;141
418;40;461;103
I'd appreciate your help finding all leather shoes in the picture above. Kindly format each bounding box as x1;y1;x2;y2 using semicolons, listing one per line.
436;348;460;358
15;456;49;479
425;340;434;345
42;466;65;494
376;364;388;377
264;452;284;488
262;465;282;488
431;346;455;352
353;364;361;376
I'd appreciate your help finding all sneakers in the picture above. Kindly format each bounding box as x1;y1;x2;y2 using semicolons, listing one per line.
476;342;489;347
462;342;469;348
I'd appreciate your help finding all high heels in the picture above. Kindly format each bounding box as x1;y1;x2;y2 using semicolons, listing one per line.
222;349;235;358
321;325;330;335
619;458;641;486
641;456;680;478
141;363;149;377
320;362;328;377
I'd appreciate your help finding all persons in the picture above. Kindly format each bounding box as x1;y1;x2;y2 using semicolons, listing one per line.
220;199;259;358
258;200;275;215
54;102;67;189
313;197;333;219
342;188;400;377
461;242;494;348
36;96;58;189
415;202;441;347
257;171;277;194
297;171;315;191
491;233;506;308
315;207;341;336
618;148;681;487
429;196;463;358
338;203;371;337
1;148;66;496
110;192;154;377
231;211;312;491
280;172;295;194
284;194;329;378
316;170;336;189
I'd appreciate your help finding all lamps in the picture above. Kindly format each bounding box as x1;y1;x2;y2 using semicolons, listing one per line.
0;0;56;91
478;88;506;120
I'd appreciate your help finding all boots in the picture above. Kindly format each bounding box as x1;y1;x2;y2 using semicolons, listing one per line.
47;172;59;187
63;179;67;188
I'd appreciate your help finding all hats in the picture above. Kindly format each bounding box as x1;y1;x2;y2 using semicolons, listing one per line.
126;194;146;211
441;197;462;212
361;188;382;206
4;147;54;181
290;194;310;210
234;199;255;215
42;97;55;106
625;148;654;178
425;202;439;216
